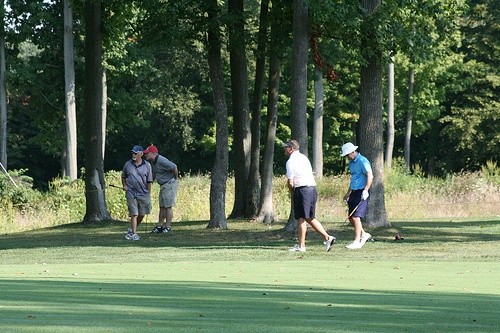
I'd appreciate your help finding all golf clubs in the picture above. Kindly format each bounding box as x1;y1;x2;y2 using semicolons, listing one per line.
109;184;124;190
145;214;148;235
333;199;363;236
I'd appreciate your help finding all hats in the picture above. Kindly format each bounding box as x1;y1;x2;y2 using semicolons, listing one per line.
340;142;358;156
142;145;158;153
281;140;299;150
130;145;144;153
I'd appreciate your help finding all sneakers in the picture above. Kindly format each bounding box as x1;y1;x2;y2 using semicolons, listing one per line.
361;233;374;246
289;244;306;252
345;240;362;248
322;235;336;251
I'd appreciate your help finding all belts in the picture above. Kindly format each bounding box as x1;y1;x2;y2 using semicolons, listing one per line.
352;190;361;193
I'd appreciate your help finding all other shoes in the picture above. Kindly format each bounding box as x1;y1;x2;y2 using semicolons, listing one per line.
152;225;167;232
132;233;140;241
124;228;133;240
162;226;171;233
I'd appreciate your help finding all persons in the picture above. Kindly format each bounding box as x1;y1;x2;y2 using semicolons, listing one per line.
341;143;373;249
285;140;336;251
121;146;153;240
144;146;178;233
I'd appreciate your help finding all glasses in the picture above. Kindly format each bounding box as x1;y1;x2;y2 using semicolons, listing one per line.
133;152;137;154
